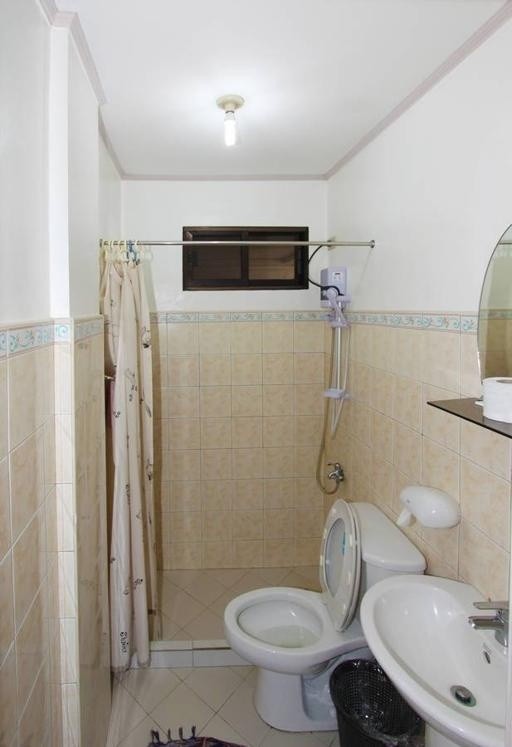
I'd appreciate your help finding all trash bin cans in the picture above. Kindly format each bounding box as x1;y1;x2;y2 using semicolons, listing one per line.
330;659;421;747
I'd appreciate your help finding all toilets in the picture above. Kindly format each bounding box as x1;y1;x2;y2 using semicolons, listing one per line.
223;498;427;732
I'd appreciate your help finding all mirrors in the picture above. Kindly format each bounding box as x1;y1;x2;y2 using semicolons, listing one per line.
476;222;512;387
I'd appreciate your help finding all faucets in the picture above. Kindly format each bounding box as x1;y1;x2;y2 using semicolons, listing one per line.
467;600;509;648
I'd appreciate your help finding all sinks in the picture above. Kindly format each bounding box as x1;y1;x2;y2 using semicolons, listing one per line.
360;573;511;747
